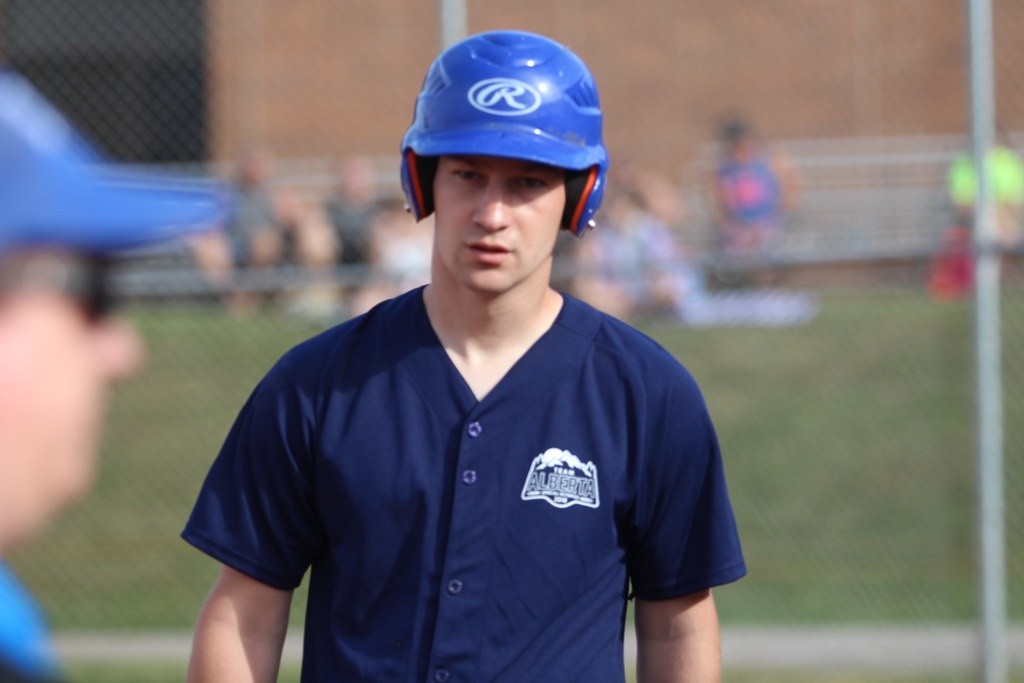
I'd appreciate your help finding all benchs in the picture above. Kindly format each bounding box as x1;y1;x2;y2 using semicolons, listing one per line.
677;136;977;276
105;159;404;295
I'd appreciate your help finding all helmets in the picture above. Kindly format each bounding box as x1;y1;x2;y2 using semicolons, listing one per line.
398;29;608;239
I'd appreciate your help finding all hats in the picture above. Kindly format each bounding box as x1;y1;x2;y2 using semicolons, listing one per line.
0;68;235;247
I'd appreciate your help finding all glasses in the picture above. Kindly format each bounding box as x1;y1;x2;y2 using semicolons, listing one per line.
0;247;112;319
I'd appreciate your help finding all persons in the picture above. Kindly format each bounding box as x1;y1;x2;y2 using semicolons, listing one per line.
0;76;226;683
180;31;747;683
949;125;1024;284
572;121;799;322
182;152;436;312
